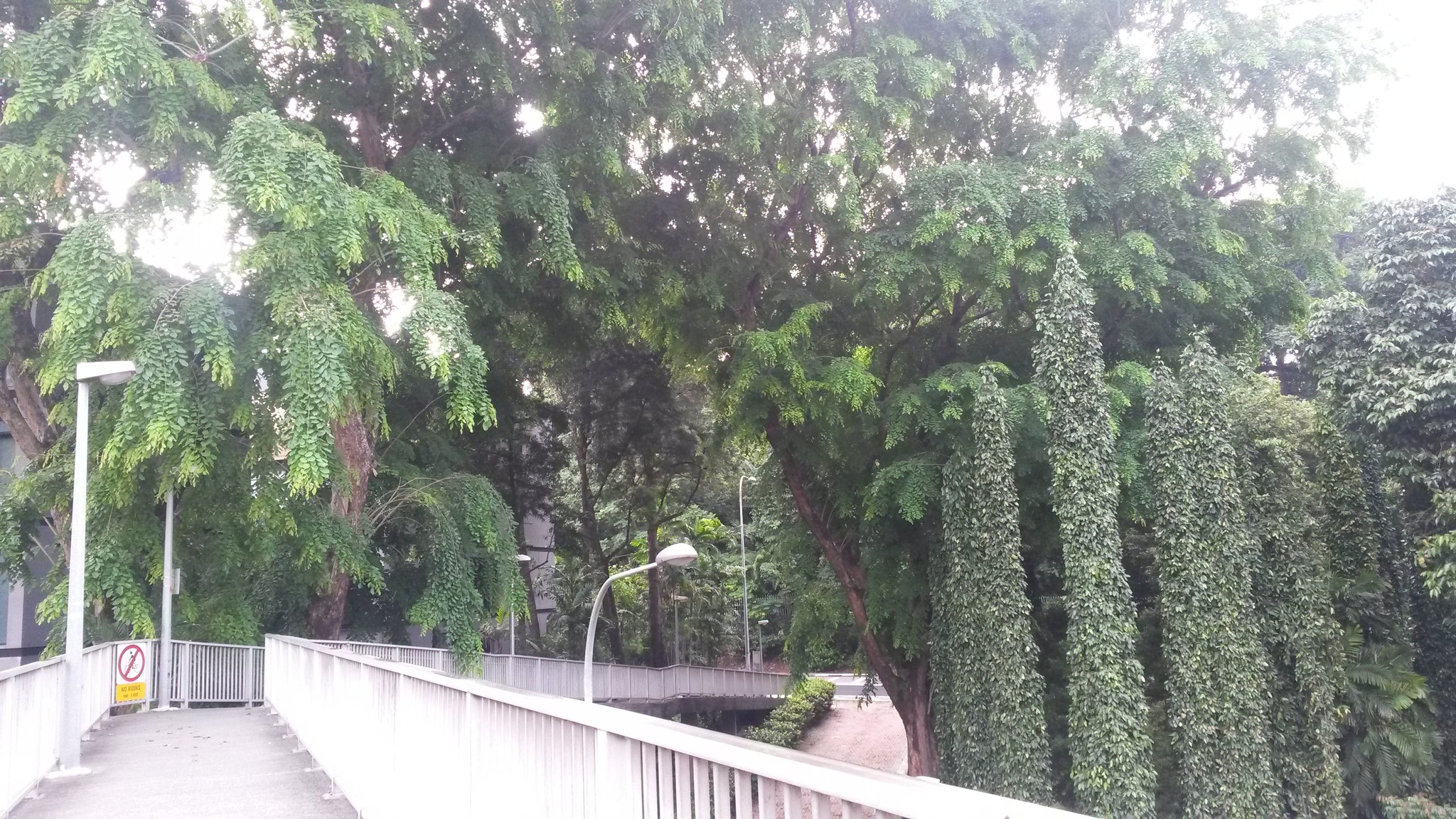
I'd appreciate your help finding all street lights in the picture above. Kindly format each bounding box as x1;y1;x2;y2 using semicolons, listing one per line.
508;554;531;654
64;359;137;773
738;475;757;672
584;543;699;703
